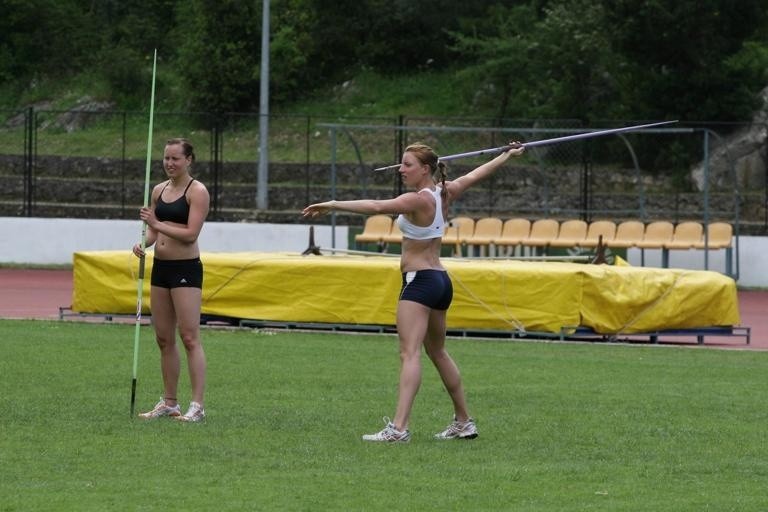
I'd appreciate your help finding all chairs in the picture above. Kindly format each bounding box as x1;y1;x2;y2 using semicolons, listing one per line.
441;215;504;258
617;221;675;268
665;212;735;281
354;214;402;254
493;217;617;263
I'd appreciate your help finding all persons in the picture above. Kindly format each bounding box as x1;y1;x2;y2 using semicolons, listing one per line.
133;138;210;424
303;140;526;443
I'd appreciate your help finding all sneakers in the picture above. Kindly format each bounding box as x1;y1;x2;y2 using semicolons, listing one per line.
175;401;207;423
361;418;410;444
138;398;183;419
433;413;479;440
